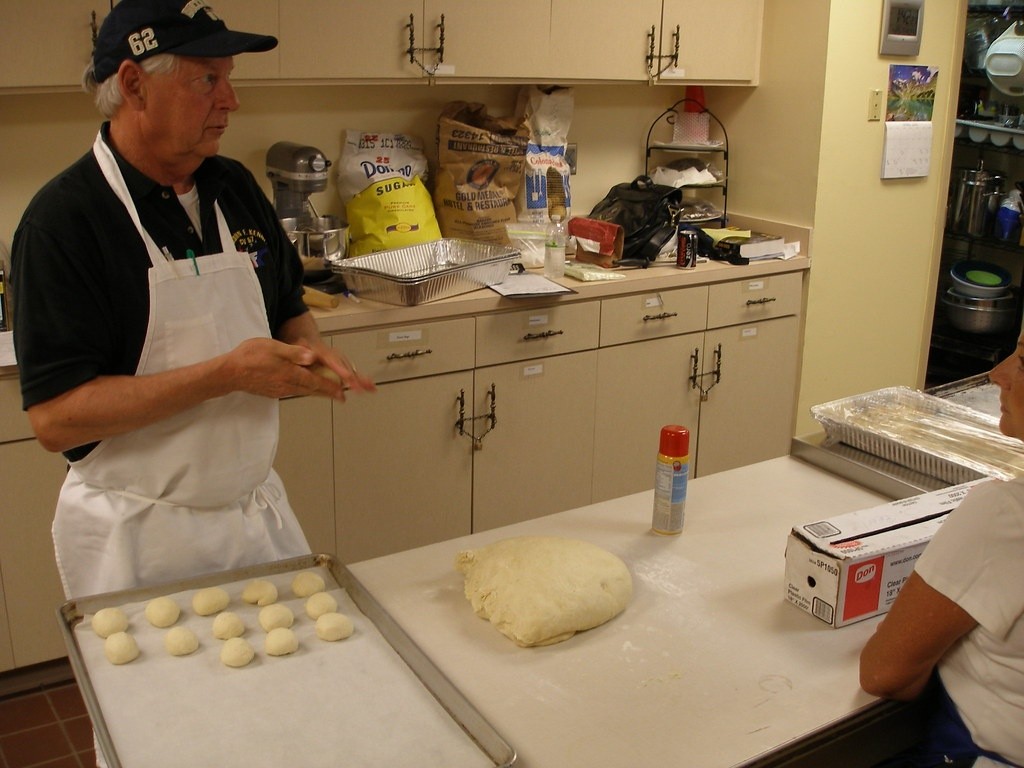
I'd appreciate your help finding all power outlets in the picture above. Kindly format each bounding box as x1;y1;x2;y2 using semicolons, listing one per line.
566;143;577;176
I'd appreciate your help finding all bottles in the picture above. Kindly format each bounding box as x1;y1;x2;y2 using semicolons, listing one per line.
544;215;565;277
651;426;691;535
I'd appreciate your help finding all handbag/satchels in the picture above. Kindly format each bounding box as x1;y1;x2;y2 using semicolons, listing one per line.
589;174;683;269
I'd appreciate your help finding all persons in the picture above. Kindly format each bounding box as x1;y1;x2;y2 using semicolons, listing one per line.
10;0;382;768
859;326;1024;768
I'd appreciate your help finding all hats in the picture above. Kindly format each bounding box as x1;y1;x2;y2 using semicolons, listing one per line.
93;0;279;84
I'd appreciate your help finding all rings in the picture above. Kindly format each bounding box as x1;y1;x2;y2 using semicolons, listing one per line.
350;366;359;376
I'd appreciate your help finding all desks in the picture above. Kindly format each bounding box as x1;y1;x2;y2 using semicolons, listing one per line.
319;379;1015;768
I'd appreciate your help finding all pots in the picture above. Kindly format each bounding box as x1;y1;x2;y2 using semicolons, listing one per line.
948;159;1008;238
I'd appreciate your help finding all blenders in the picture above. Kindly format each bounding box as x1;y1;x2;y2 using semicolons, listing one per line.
262;141;347;295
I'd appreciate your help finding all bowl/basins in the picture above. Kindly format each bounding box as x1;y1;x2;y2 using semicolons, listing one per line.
943;260;1018;336
277;216;353;273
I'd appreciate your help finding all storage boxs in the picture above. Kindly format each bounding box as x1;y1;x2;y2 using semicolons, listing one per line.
784;472;1001;625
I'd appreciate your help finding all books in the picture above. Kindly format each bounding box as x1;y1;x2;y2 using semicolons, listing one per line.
696;228;785;260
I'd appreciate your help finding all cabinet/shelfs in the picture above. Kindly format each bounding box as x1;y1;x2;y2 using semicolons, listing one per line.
330;300;602;567
0;0;279;97
587;269;806;504
277;0;553;85
551;0;767;88
0;334;332;671
929;3;1024;370
